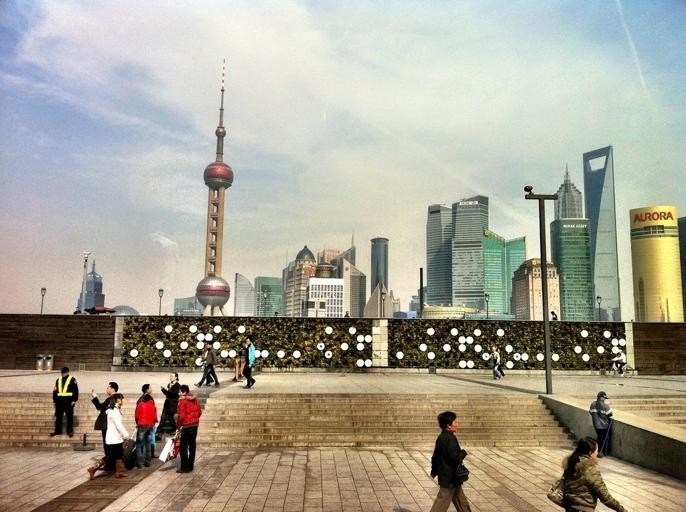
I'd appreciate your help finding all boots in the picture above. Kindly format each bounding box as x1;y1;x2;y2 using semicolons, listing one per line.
87;458;130;479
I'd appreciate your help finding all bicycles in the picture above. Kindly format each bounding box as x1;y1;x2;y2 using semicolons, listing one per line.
605;361;634;378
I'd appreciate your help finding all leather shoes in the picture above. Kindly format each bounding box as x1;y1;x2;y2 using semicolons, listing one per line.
67;432;73;437
50;432;61;436
243;379;255;389
176;469;189;473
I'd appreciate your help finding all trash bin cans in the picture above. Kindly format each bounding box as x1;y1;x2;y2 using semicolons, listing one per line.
429;360;436;374
35;353;54;370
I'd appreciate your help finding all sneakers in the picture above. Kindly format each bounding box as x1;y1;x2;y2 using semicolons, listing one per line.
194;380;220;387
136;462;150;468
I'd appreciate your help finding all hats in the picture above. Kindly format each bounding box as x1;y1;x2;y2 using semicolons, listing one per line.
597;391;608;399
61;367;69;373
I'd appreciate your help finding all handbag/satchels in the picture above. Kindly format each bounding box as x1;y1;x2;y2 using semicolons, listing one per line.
547;480;566;509
159;437;181;463
456;464;469;481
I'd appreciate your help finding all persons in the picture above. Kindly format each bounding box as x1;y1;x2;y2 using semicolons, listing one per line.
489;345;506;380
430;412;472;512
50;367;202;480
611;351;627;374
344;311;350;317
550;311;558;320
273;311;279;317
194;337;256;389
547;391;628;512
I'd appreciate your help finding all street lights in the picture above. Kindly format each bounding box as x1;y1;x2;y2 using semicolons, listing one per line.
159;288;163;316
41;288;46;314
597;295;601;323
485;294;489;319
263;290;268;316
379;292;386;317
